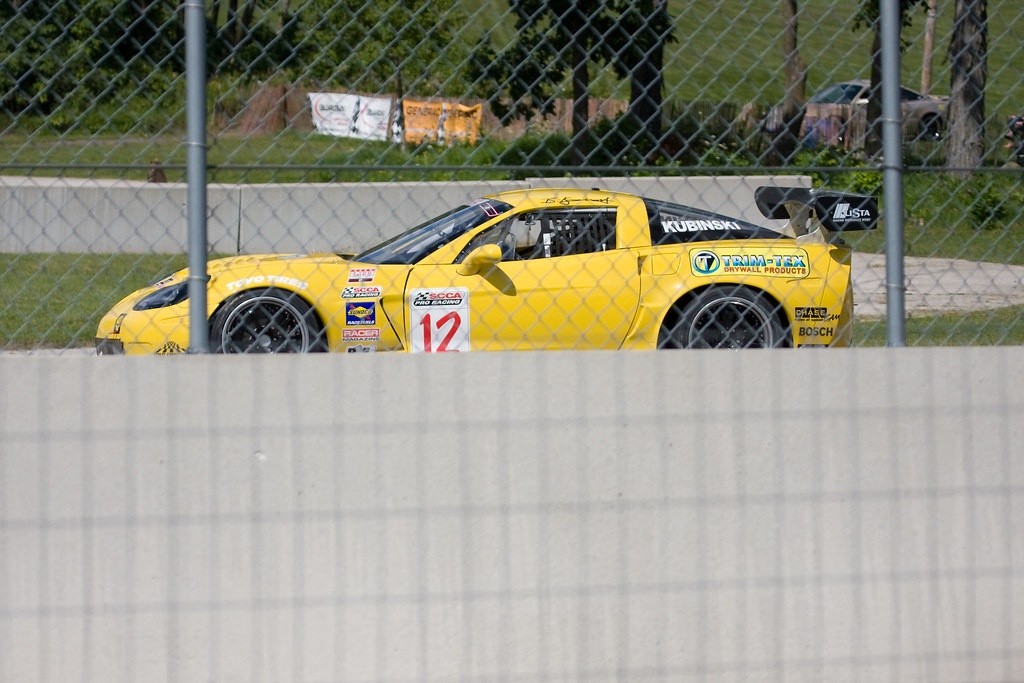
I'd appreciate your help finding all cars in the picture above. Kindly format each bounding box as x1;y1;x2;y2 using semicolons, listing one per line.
765;78;949;153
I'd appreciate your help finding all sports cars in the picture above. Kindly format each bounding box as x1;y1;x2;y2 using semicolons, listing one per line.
95;189;880;357
